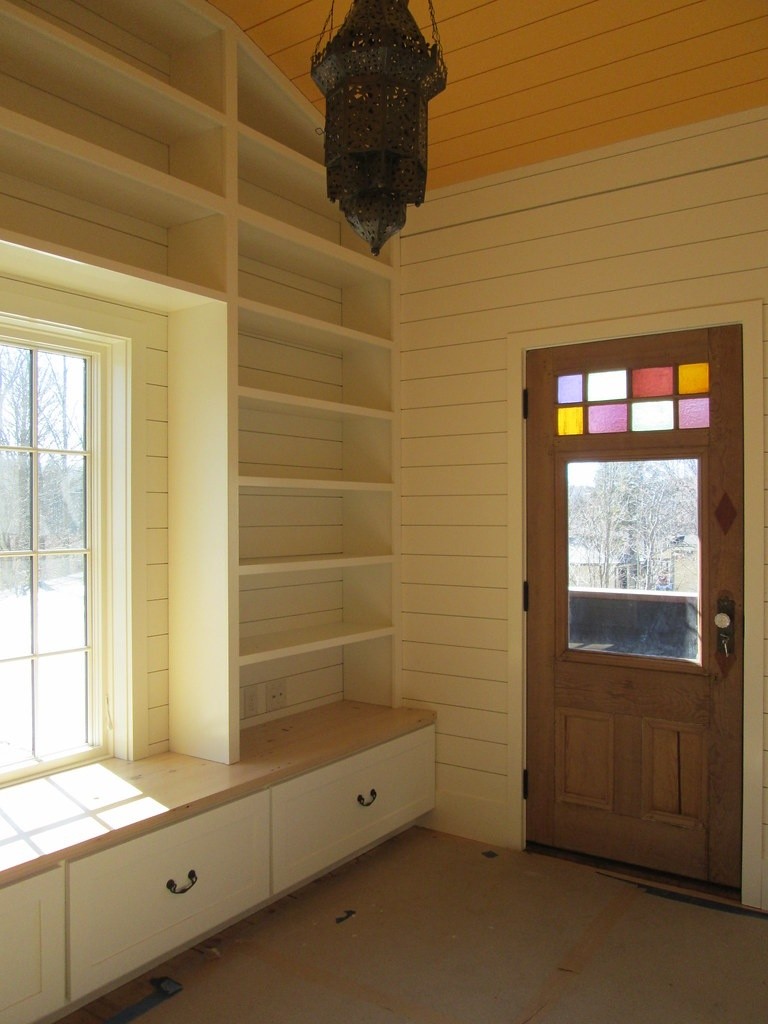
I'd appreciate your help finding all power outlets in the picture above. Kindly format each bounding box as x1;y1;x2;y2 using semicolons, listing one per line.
265;679;287;713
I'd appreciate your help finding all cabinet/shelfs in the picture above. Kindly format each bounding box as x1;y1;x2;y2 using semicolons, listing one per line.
1;0;403;764
0;698;435;1024
